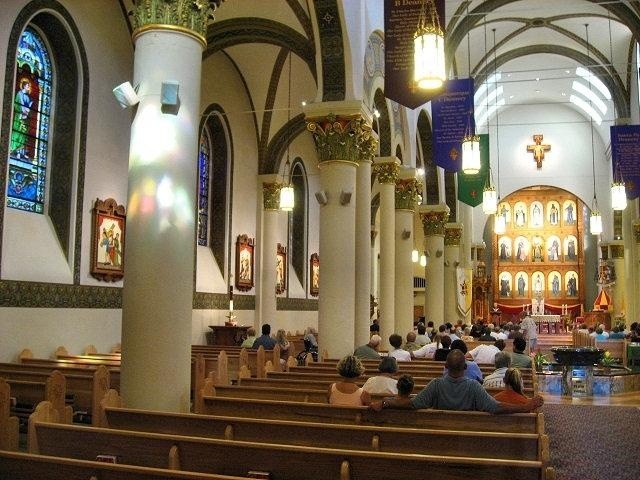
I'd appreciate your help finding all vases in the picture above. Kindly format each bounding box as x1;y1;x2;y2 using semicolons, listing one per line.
604;367;611;373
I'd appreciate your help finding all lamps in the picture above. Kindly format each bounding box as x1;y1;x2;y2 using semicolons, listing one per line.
610;11;627;211
413;0;506;234
584;24;603;235
280;52;296;212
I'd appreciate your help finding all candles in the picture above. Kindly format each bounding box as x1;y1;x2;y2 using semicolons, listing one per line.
230;300;233;312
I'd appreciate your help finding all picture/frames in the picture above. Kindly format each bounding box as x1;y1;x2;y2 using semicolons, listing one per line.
235;234;319;297
91;198;126;282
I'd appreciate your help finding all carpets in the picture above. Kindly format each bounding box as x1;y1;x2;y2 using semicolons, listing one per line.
542;405;640;480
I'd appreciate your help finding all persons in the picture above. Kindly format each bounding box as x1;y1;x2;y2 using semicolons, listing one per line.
500;203;576;298
578;321;640;346
100;228;121;267
242;323;287;350
9;81;35;162
356;303;544;368
327;340;545;416
296;334;318;363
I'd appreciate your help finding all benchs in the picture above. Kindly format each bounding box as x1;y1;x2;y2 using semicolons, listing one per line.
0;330;556;480
572;330;631;366
